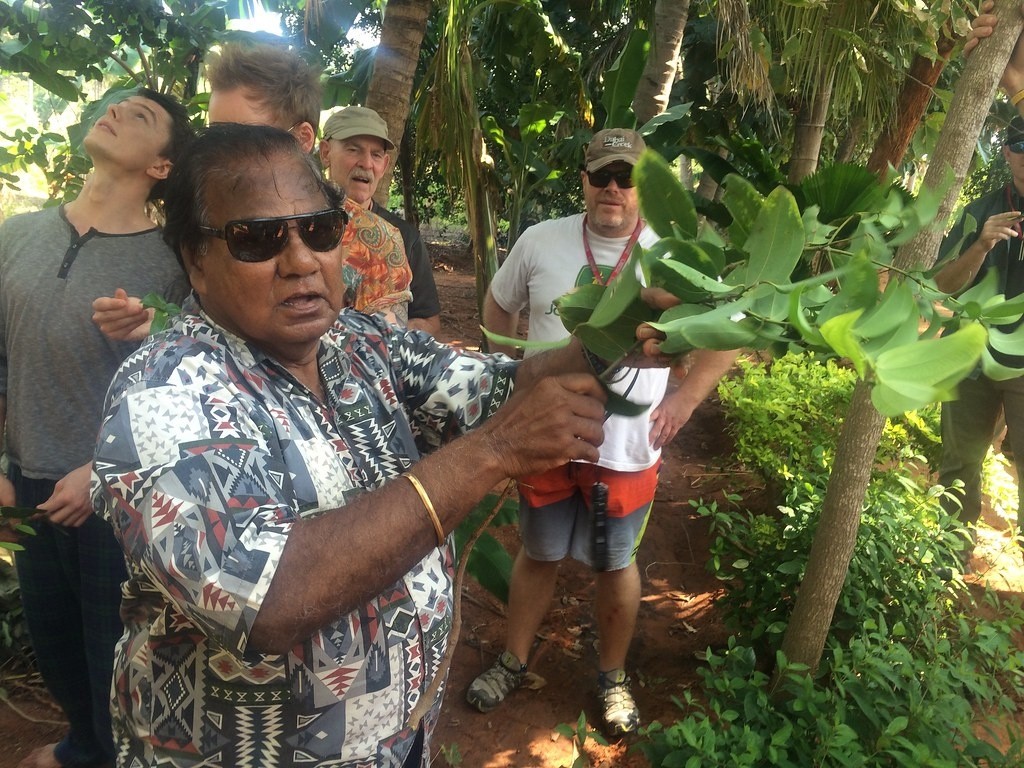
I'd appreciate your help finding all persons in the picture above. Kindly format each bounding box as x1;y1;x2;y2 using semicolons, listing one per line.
91;121;686;767
91;28;412;356
320;103;441;337
0;88;199;768
934;118;1024;581
963;0;1024;117
464;126;744;736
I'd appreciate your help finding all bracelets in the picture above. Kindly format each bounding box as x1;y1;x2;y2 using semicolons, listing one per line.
400;471;446;549
1008;88;1024;109
580;344;625;377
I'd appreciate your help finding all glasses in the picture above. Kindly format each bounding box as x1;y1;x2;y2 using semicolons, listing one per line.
197;204;351;262
587;170;634;189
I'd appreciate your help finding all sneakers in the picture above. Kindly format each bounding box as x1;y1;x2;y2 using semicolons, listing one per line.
466;652;528;713
597;665;640;735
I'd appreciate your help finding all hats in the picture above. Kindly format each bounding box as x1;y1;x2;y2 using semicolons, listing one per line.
585;128;646;174
322;106;395;150
